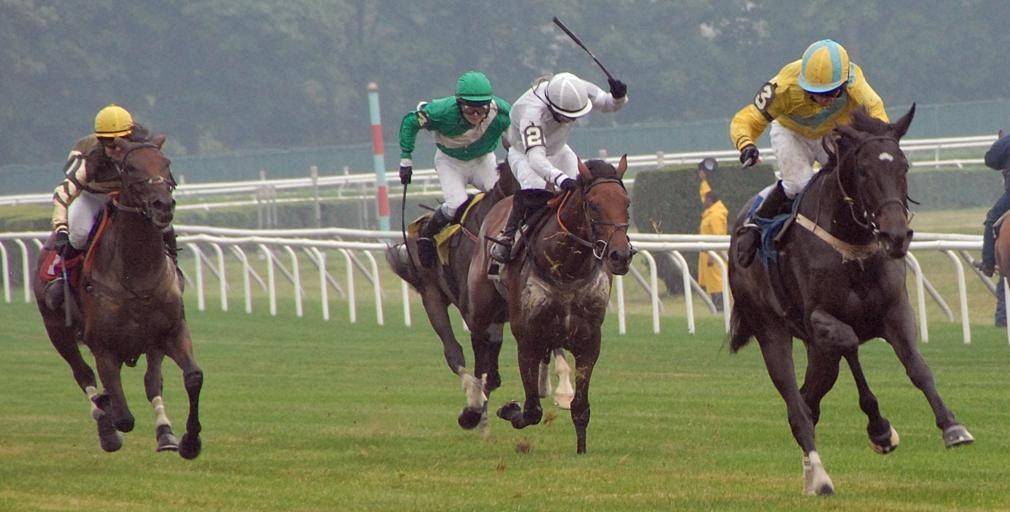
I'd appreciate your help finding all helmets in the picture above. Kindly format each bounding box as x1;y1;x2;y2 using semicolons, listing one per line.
455;71;492;107
95;104;134;138
798;39;850;94
544;72;593;118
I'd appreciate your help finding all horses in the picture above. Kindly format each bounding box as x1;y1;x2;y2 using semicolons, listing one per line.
729;101;974;496
384;135;575;430
458;153;636;454
34;122;203;460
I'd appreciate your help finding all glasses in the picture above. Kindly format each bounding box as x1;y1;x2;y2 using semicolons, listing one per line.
461;104;492;114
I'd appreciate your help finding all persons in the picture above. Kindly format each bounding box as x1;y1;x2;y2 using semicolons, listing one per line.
971;134;1010;276
729;38;890;268
43;102;185;311
400;71;513;268
490;73;629;264
696;166;729;310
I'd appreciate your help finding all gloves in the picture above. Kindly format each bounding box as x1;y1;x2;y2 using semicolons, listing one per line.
740;146;759;168
608;77;627;100
561;178;577;193
53;223;71;256
399;165;412;184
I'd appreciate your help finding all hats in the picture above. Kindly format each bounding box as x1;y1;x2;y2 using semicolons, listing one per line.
697;158;719;173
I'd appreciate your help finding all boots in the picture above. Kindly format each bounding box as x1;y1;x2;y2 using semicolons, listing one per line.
44;241;83;311
491;189;545;262
735;180;799;269
416;208;451;270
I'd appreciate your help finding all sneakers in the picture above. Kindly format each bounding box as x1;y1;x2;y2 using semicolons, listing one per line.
973;260;995;278
